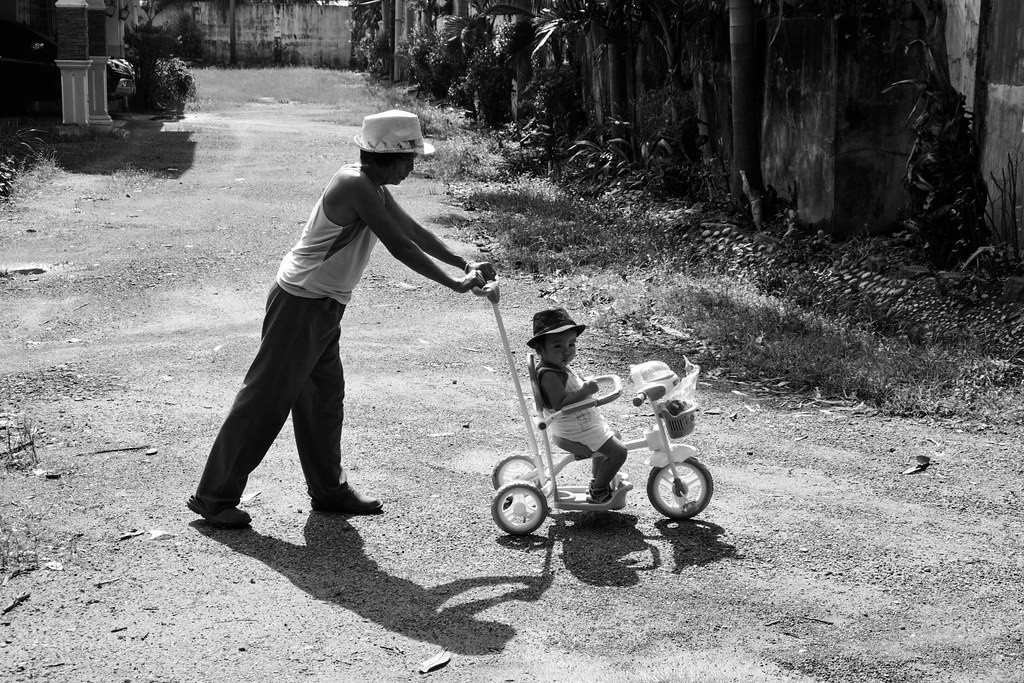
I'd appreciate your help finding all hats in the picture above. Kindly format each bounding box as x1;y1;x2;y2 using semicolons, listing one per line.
353;109;435;155
526;307;586;349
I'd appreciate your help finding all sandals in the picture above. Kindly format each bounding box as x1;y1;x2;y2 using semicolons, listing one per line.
585;478;615;504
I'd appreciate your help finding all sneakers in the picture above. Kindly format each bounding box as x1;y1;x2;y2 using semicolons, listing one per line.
311;481;383;513
187;495;252;529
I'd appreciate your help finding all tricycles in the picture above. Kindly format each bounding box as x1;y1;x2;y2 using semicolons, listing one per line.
464;260;716;536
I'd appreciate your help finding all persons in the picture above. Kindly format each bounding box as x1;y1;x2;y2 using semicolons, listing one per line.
187;108;496;526
527;307;628;510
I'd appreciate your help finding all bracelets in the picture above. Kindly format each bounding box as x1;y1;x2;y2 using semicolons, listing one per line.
465;261;475;273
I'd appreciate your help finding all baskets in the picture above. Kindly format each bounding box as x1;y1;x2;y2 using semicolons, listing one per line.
660;399;698;439
